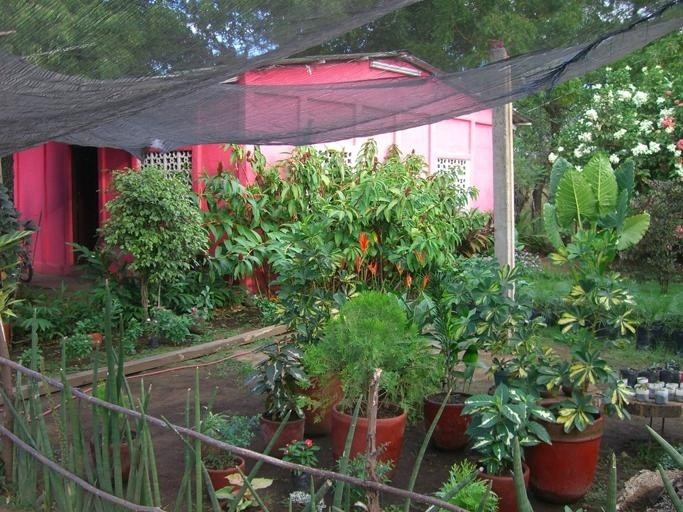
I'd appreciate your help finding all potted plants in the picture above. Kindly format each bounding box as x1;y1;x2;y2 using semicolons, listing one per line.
0;230;36;346
